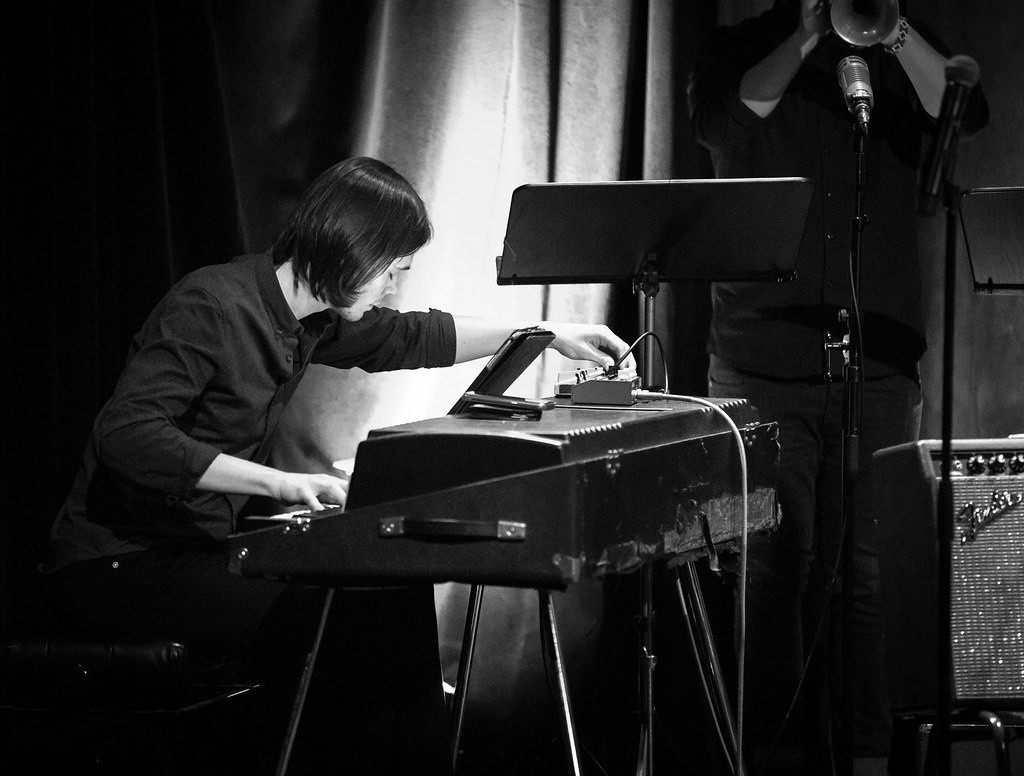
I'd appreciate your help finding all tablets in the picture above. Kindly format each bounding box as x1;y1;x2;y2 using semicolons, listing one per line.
448;328;547;414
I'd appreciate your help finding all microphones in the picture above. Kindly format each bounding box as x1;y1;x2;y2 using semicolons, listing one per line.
835;56;876;136
919;54;980;216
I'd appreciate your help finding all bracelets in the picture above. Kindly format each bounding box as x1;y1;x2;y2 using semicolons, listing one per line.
883;13;909;55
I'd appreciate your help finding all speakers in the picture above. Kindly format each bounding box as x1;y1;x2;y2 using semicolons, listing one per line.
887;706;1024;776
868;434;1024;713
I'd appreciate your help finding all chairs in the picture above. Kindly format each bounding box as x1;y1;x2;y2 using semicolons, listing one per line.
0;463;268;776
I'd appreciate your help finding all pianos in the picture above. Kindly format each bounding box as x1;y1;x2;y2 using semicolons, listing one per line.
230;327;788;775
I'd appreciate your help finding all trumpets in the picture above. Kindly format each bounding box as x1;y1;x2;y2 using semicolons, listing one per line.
830;1;902;48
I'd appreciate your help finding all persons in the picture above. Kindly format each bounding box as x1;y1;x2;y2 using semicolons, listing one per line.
687;1;992;774
49;156;638;776
